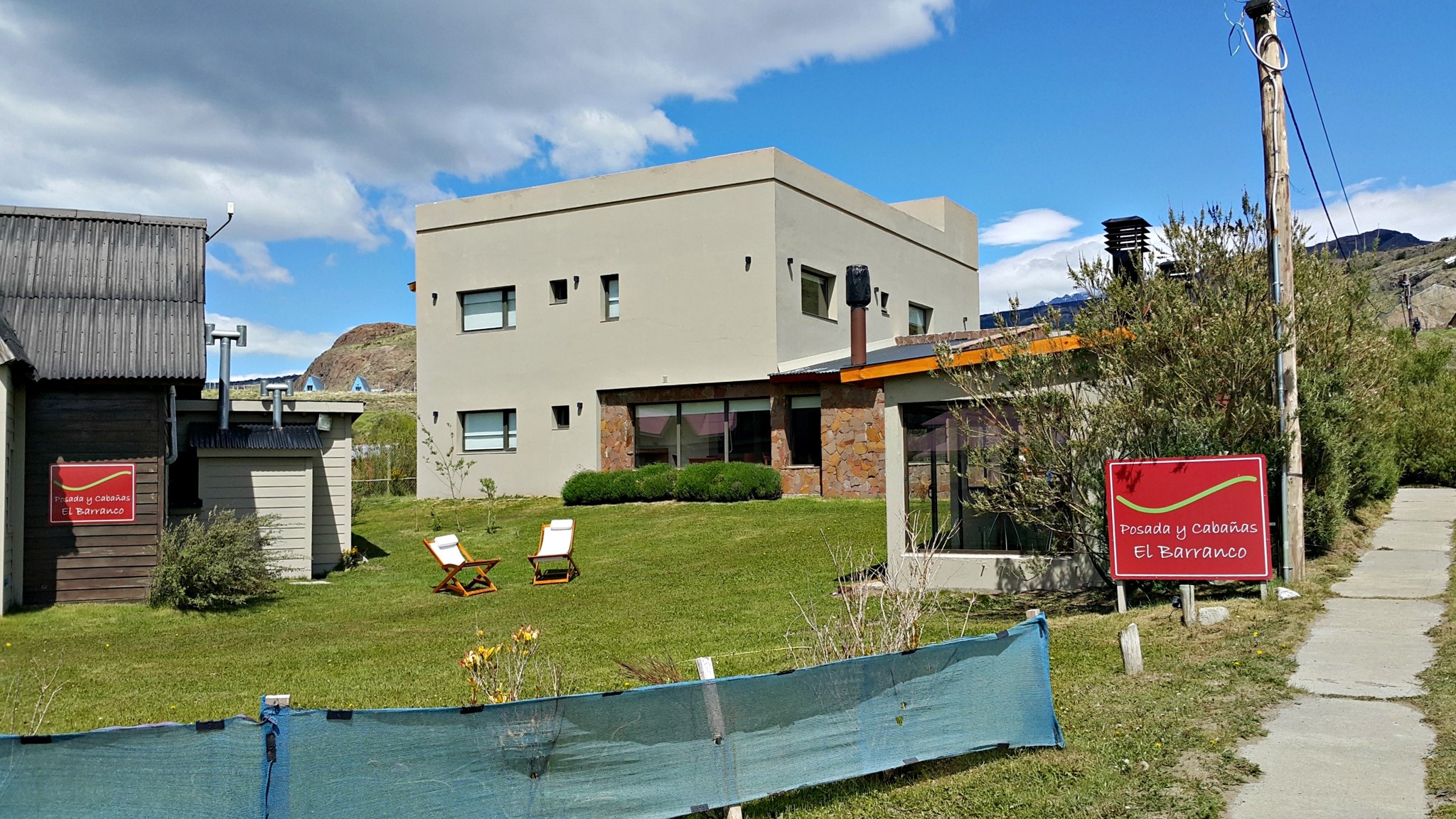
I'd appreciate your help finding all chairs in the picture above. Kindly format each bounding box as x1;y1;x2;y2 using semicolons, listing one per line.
526;519;581;586
672;455;677;467
422;534;501;599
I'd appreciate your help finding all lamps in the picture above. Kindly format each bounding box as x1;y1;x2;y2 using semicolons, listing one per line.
316;412;332;432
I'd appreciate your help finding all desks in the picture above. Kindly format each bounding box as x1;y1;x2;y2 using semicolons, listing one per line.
687;457;723;463
763;456;770;465
